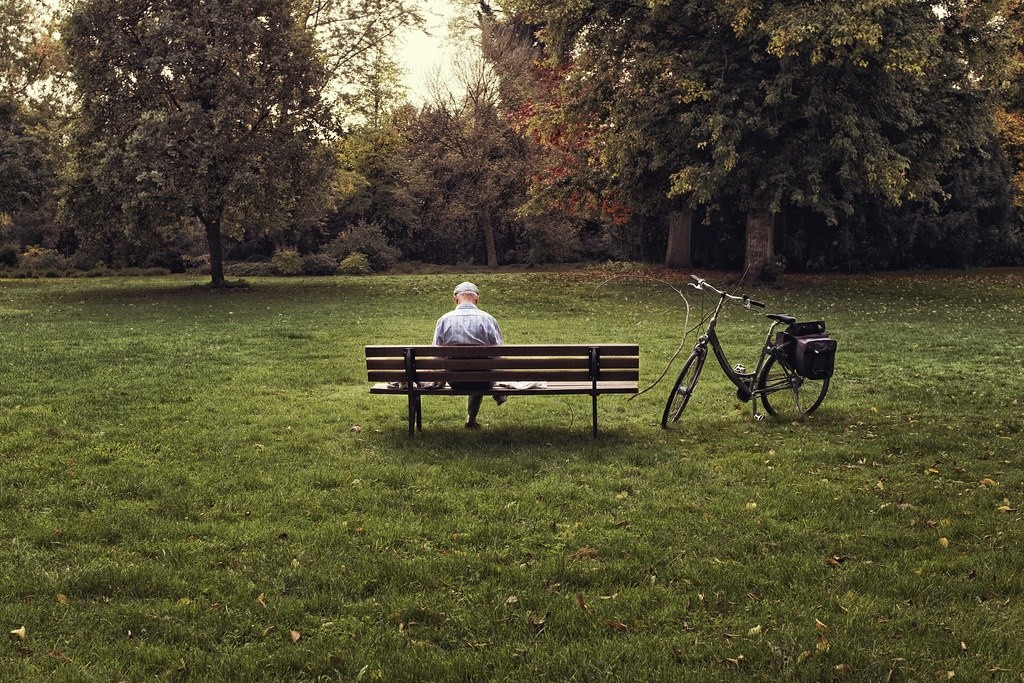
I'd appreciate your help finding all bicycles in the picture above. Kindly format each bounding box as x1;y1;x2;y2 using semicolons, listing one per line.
658;273;838;431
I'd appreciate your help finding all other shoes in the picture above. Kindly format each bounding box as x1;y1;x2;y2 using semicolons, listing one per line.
465;421;481;430
493;395;507;405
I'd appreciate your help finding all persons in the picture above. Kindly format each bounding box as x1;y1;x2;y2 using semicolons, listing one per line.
432;282;507;429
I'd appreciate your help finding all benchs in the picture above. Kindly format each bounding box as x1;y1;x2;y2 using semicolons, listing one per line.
364;344;639;437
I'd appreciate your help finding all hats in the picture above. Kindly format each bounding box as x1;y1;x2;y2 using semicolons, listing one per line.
454;282;479;298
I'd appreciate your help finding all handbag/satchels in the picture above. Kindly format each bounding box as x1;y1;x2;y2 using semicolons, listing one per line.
776;321;837;380
